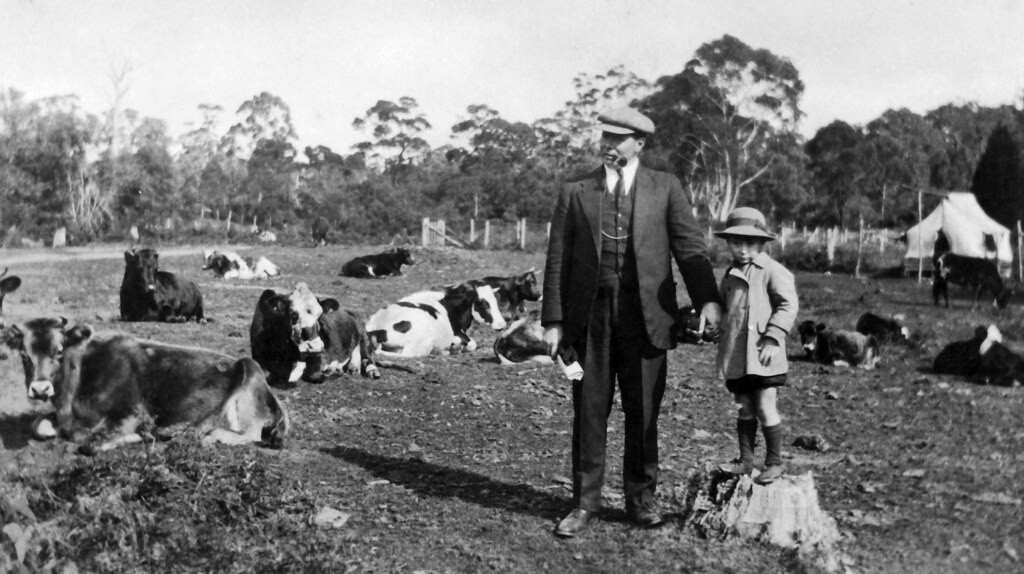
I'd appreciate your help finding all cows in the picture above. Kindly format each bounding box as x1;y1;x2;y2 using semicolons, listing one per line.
338;245;415;280
200;245;281;283
482;265;560;366
793;319;880;369
249;282;384;389
7;314;287;457
312;215;331;247
930;252;1024;388
360;279;508;374
120;247;208;325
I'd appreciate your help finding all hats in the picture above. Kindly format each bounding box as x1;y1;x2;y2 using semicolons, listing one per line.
597;105;656;134
713;207;776;241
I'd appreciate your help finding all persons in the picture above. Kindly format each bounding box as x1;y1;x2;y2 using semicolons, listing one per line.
541;108;724;538
705;207;799;485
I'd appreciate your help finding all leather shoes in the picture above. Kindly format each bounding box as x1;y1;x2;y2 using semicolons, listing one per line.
557;508;595;536
627;504;661;527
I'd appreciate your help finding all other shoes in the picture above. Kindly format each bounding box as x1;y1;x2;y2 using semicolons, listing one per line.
718;456;753;473
759;462;786;483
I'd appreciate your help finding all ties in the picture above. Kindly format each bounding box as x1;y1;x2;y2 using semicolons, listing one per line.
615;168;625;197
